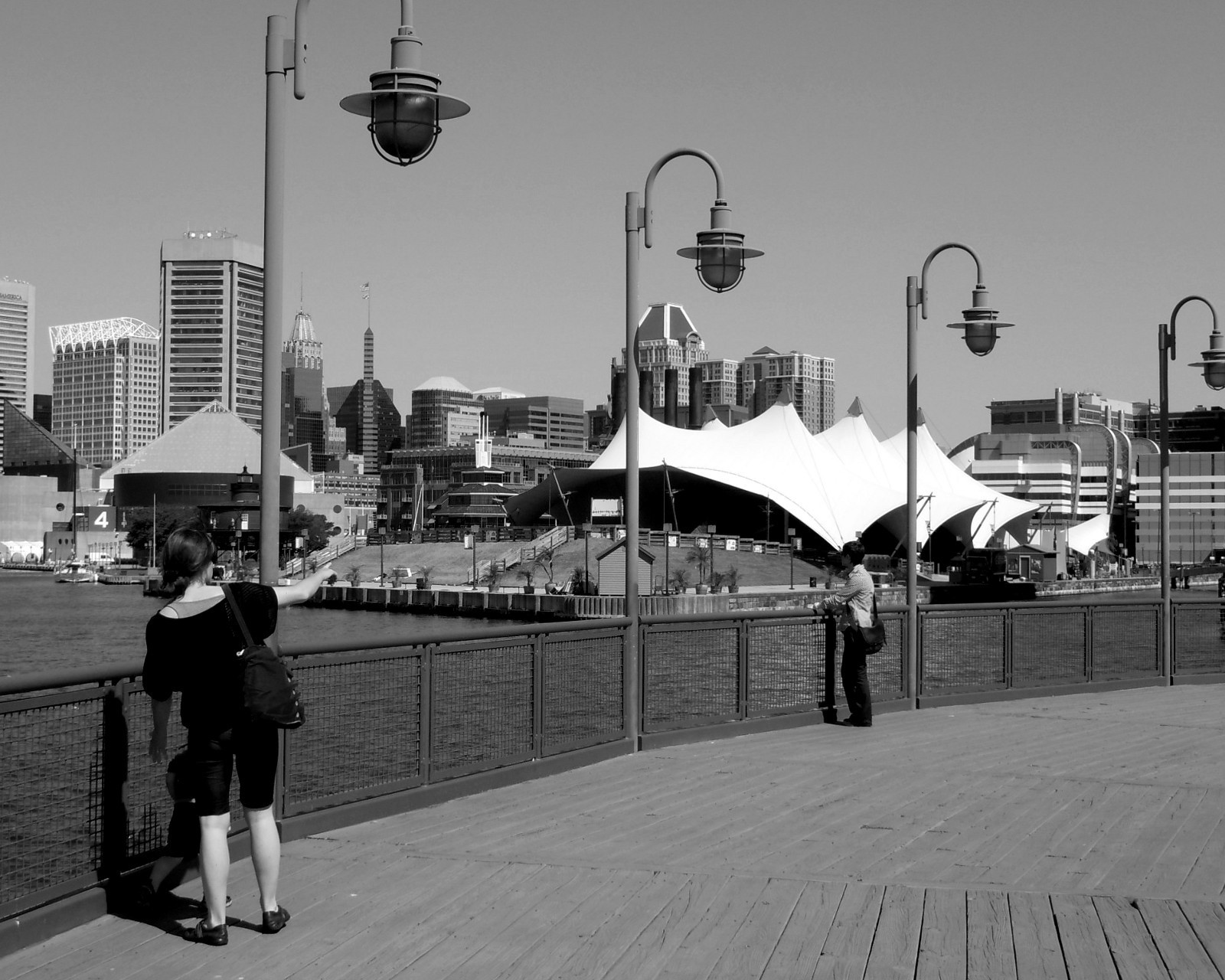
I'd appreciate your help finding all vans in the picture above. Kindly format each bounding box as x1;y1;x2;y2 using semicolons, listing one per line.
84;552;115;565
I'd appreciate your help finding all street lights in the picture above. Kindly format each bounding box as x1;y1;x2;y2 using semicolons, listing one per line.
788;528;796;589
625;149;767;751
583;524;592;595
906;242;1018;710
379;527;386;587
472;524;480;591
1159;294;1225;686
1188;513;1199;566
259;1;472;636
231;542;236;565
708;525;717;594
664;524;672;597
144;541;156;567
301;529;308;579
236;529;241;558
284;543;292;565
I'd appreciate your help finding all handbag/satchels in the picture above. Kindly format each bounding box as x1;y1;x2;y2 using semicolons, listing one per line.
856;618;887;655
222;583;304;730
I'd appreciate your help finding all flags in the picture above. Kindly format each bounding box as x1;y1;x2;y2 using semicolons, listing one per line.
361;282;369;299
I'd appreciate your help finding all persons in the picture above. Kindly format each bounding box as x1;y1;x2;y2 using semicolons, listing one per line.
225;559;234;580
142;526;290;946
813;541;872;726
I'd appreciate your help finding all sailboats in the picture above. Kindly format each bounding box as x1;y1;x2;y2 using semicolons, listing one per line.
52;447;97;582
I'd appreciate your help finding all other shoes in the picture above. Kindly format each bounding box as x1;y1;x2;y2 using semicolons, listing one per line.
201;895;231;910
844;716;872;728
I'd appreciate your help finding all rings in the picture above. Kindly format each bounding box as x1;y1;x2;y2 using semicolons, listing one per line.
156;749;160;753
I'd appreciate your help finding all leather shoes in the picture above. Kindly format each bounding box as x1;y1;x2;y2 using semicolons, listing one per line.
194;920;227;946
262;904;290;934
